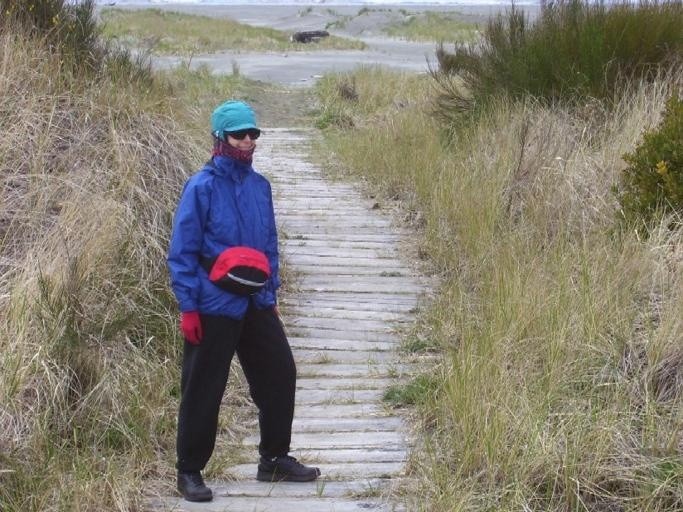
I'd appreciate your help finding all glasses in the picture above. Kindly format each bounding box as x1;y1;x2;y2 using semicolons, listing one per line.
225;128;260;139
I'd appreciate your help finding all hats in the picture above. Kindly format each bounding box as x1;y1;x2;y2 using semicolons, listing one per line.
211;100;261;141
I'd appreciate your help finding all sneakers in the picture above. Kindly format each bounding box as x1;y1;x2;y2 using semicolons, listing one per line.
177;471;213;502
257;455;321;482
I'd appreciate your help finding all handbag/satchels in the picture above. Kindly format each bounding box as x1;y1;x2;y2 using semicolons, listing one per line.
208;246;269;296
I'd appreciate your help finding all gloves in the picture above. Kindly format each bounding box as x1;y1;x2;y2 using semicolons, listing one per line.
181;311;202;345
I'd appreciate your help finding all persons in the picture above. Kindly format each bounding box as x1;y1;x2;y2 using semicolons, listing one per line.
165;99;321;502
